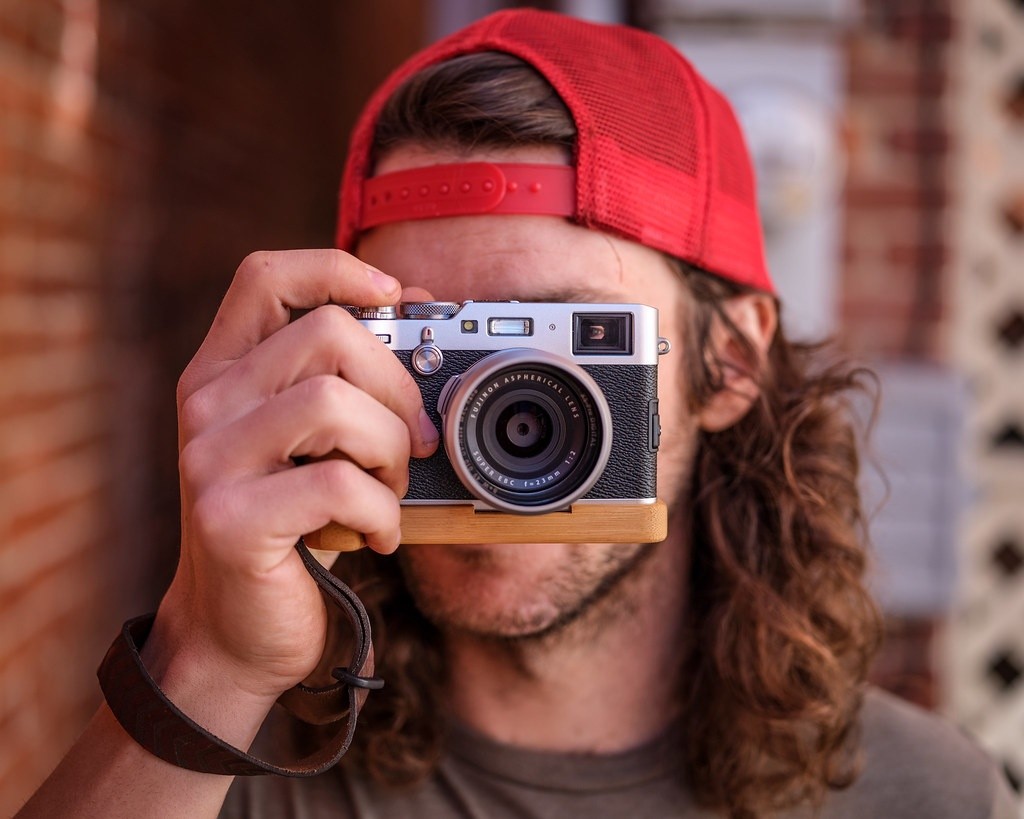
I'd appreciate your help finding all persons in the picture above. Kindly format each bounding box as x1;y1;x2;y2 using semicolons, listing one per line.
8;9;1024;819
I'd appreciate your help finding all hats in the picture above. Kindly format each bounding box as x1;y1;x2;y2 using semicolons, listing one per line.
337;9;779;299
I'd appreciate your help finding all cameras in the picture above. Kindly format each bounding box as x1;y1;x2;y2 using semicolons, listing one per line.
299;298;670;552
